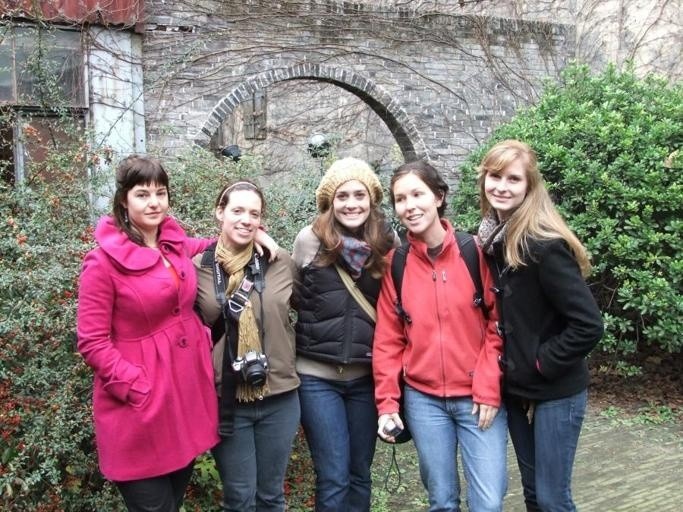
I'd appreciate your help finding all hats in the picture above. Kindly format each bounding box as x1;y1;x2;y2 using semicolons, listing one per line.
316;157;384;214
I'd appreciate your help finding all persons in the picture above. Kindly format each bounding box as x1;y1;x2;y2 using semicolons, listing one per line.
290;159;403;512
183;176;303;512
75;153;281;512
471;137;604;512
369;160;511;511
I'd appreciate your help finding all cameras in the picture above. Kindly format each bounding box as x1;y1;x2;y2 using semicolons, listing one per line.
232;352;269;387
384;419;402;438
